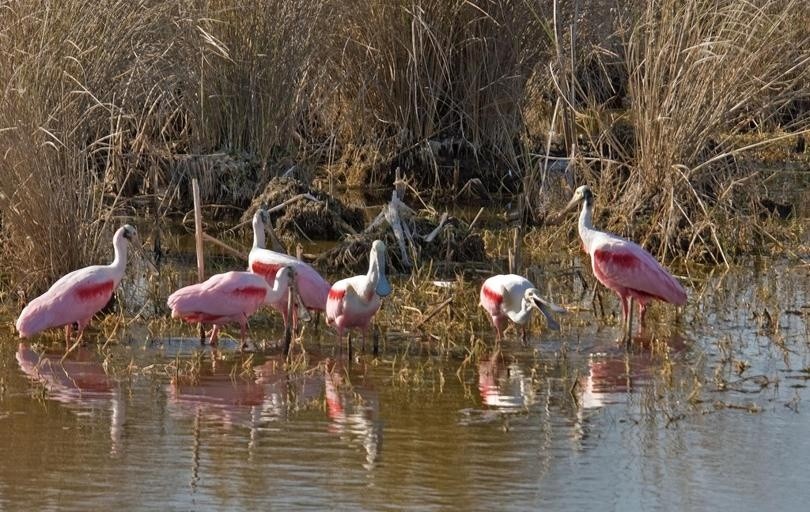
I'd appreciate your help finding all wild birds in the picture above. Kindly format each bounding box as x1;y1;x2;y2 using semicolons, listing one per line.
167;264;312;349
16;224;159;350
325;239;390;346
556;184;688;323
247;208;332;329
479;273;567;343
